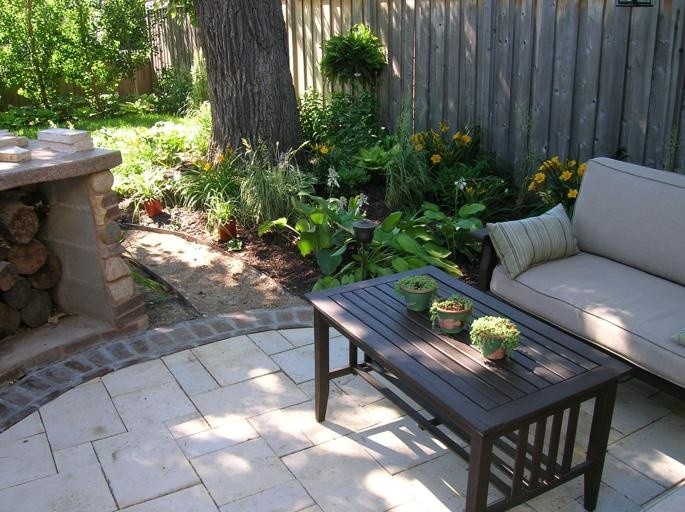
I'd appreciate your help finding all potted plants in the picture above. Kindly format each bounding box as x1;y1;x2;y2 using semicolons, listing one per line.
124;173;177;225
203;192;246;246
394;273;439;312
469;314;522;362
429;293;475;335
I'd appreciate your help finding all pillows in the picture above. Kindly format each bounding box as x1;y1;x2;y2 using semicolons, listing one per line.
485;202;581;281
669;326;685;348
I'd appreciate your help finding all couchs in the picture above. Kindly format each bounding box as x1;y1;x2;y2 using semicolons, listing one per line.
489;156;685;388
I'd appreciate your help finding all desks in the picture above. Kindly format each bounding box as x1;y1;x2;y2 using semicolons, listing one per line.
300;264;634;511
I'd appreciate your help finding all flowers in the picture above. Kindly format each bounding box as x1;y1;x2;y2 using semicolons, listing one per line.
524;156;586;205
405;121;474;166
187;150;224;175
306;142;334;169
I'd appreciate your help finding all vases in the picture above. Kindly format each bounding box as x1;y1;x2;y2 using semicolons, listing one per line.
353;221;377;243
